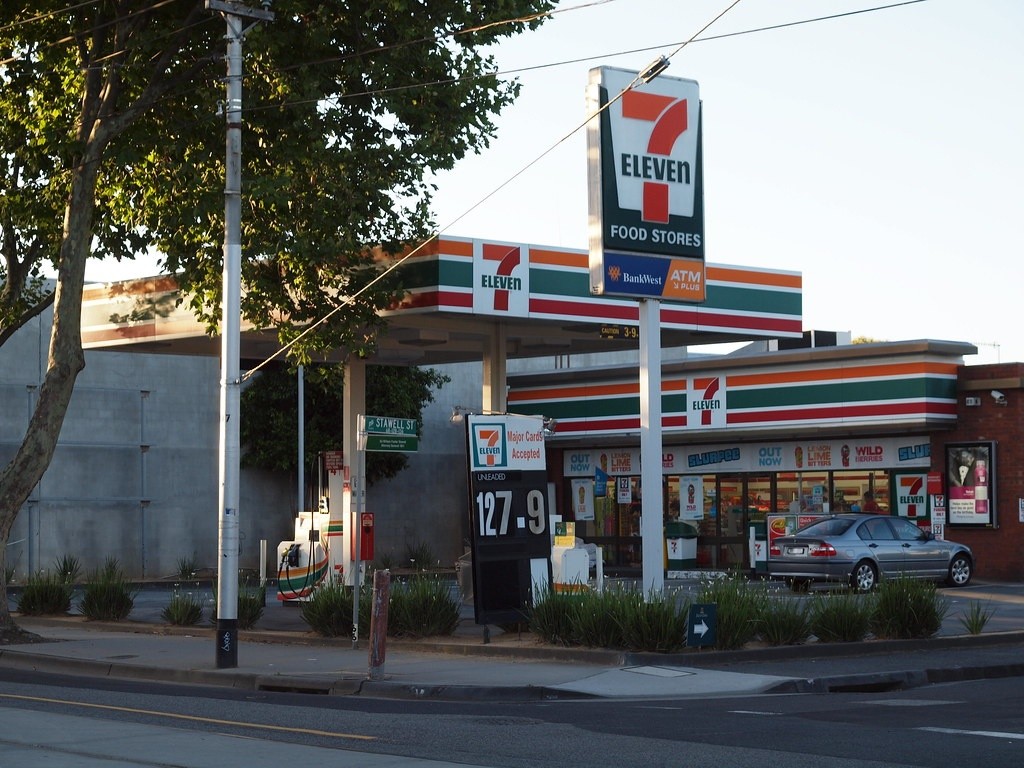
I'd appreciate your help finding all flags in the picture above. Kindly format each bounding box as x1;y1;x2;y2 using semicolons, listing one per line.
595;467;608;496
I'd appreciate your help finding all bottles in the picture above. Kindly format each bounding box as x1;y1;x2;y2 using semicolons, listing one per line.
973;460;989;513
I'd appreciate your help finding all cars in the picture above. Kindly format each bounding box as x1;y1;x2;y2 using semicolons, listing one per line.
766;514;974;594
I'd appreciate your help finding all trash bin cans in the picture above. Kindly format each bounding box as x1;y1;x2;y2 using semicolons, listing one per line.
747;519;768;571
664;522;698;571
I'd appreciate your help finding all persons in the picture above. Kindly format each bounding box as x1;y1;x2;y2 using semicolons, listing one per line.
851;501;861;512
863;491;882;512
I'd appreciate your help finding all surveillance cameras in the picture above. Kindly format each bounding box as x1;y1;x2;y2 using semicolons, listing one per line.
990;390;1005;402
